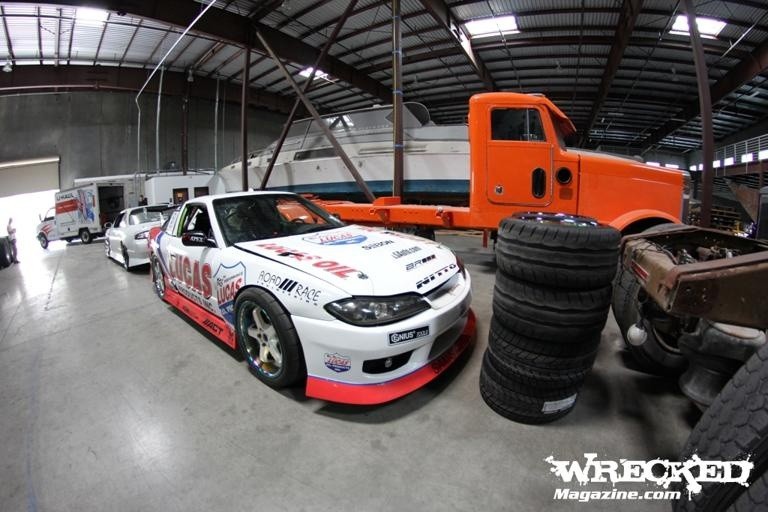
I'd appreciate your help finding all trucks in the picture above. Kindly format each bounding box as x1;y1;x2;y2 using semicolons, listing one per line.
275;93;691;242
37;182;127;248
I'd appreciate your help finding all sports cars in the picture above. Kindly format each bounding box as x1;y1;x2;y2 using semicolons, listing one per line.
151;188;476;406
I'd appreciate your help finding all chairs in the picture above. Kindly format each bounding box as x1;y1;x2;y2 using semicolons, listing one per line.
195;203;271;239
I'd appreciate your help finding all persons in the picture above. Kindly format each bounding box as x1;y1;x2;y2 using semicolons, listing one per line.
138;195;146;206
6;217;20;264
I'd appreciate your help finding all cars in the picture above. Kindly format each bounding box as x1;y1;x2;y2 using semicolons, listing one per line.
105;204;181;270
